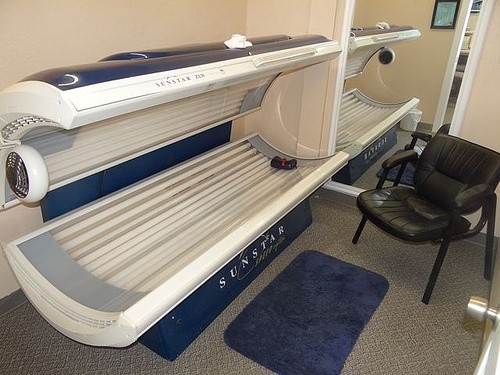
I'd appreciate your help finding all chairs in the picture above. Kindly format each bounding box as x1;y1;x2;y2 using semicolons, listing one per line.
391;123;451;187
351;131;500;305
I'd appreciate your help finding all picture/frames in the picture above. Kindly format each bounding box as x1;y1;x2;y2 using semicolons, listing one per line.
430;0;461;30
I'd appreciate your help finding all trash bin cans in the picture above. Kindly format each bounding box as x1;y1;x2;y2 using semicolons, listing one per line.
400;109;423;131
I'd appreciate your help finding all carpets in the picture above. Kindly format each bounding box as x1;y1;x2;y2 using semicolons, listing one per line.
223;249;390;375
375;150;419;187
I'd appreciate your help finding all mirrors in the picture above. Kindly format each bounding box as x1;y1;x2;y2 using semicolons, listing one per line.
322;0;495;199
